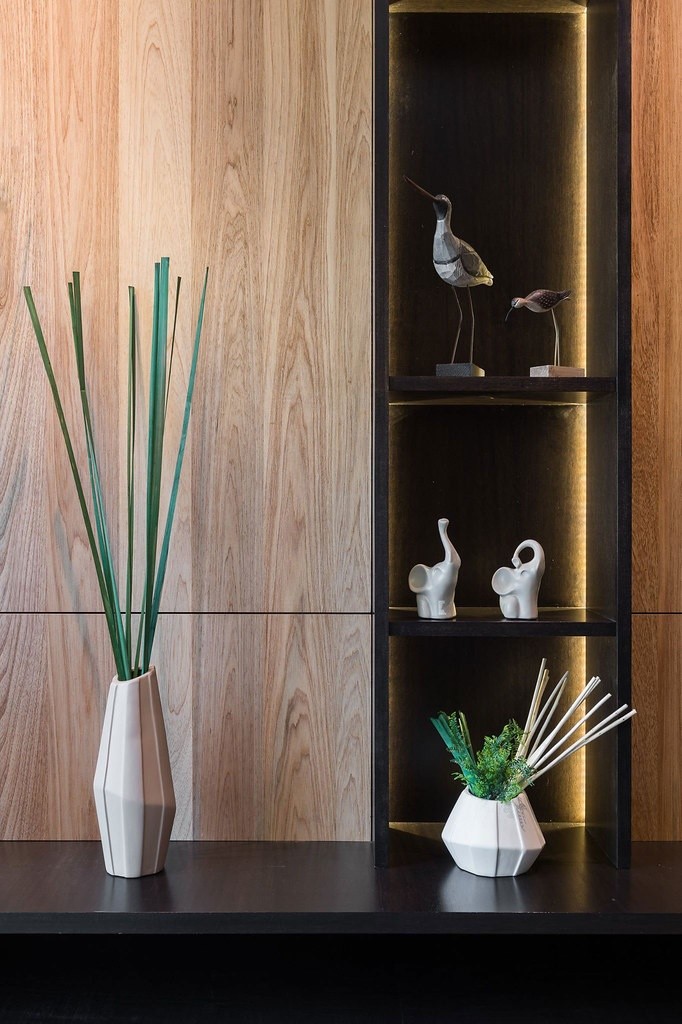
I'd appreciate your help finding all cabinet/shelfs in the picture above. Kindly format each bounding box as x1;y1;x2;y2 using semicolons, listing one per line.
368;0;632;876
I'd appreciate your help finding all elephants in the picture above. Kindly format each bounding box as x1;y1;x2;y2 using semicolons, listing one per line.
409;519;461;619
492;539;545;619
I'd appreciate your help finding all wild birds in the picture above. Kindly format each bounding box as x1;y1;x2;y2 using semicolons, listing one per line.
394;172;496;364
505;289;572;366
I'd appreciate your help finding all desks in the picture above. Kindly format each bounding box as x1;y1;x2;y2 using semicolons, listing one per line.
0;838;682;1024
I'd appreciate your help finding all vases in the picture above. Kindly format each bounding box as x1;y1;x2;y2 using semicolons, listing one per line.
92;666;178;879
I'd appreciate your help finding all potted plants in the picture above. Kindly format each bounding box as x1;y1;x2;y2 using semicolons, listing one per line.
440;711;546;877
